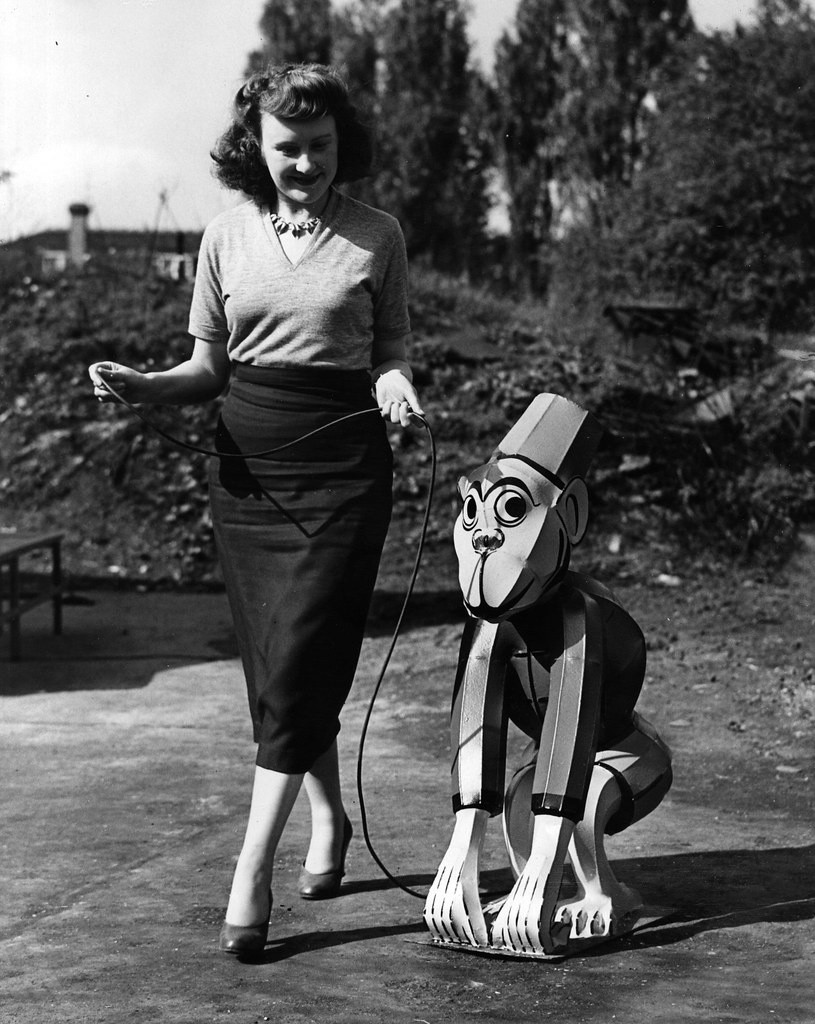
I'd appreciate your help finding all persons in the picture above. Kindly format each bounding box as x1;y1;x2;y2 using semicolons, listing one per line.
88;63;424;953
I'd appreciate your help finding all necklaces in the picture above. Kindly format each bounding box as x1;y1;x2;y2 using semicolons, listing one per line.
270;210;322;237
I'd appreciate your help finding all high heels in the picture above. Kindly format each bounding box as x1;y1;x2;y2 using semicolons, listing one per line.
217;889;275;956
296;811;352;900
